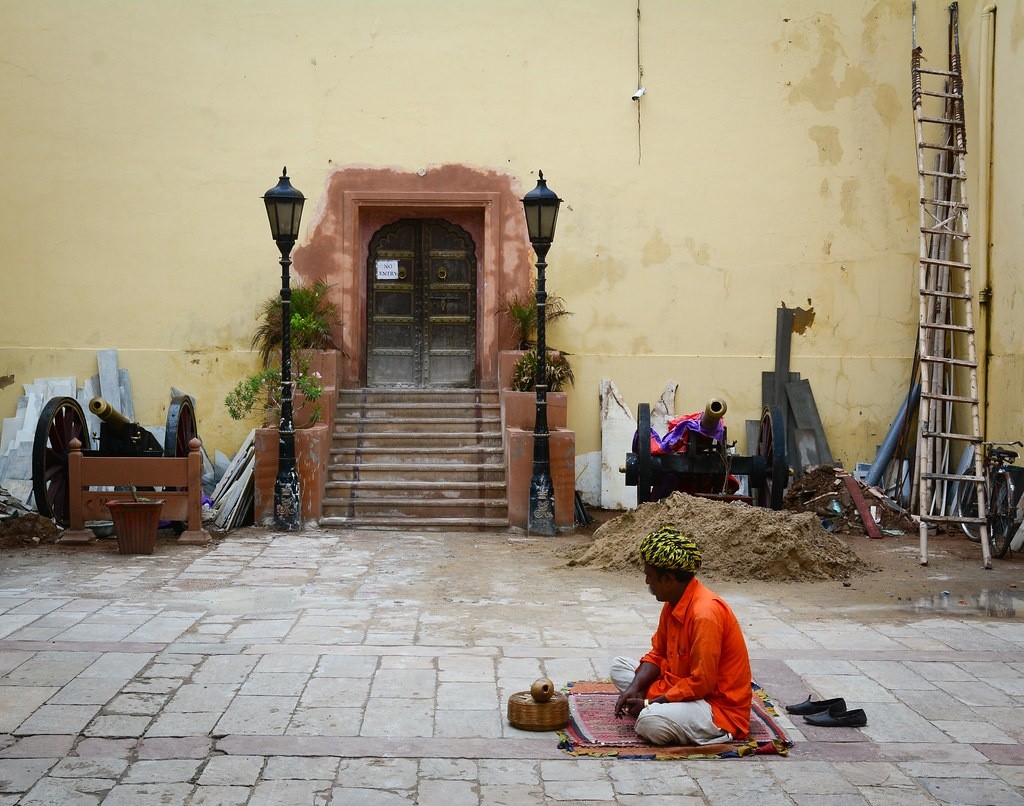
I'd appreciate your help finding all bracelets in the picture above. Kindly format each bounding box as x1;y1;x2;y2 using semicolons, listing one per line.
644;699;649;708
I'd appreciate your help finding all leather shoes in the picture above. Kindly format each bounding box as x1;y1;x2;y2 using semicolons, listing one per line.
803;706;867;727
786;695;847;713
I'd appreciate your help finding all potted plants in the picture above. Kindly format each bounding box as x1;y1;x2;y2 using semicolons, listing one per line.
695;438;756;506
105;479;166;556
494;277;575;405
250;276;346;387
500;348;575;450
225;310;330;523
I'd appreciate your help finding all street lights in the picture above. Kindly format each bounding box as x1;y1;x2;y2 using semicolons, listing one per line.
259;167;309;534
520;169;565;536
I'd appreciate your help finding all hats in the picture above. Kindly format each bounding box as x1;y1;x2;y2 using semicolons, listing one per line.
640;526;702;572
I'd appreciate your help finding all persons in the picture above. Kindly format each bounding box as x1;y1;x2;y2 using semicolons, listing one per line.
610;526;753;745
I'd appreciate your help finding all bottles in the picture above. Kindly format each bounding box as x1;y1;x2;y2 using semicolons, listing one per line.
832;499;841;512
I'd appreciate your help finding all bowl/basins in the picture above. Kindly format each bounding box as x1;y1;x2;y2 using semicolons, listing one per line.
85;520;114;537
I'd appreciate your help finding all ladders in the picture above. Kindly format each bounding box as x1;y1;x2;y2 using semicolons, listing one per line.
911;2;993;569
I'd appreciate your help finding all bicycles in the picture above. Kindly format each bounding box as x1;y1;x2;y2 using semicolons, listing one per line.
957;439;1023;558
